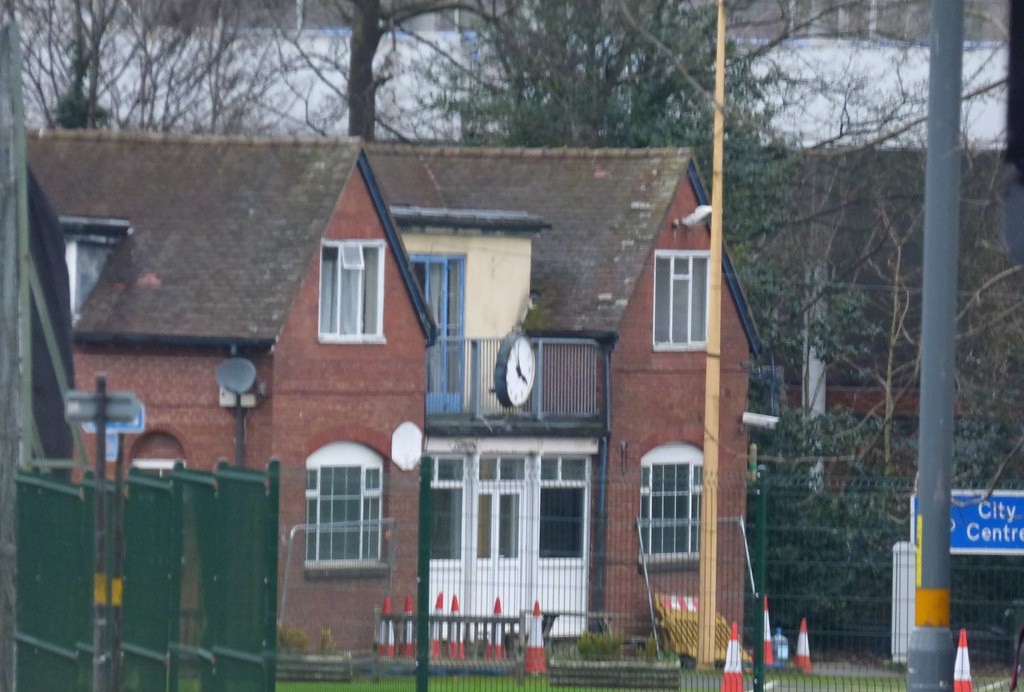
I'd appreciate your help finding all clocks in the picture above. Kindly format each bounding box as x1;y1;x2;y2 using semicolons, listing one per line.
496;330;537;410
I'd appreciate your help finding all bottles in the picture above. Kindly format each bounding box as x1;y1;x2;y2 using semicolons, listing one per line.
771;627;789;668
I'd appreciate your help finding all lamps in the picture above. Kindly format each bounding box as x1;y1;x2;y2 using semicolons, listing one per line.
672;202;713;236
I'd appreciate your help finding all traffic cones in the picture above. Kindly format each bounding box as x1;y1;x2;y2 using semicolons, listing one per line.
719;621;744;692
447;595;465;660
484;598;503;661
791;618;814;677
523;600;548;676
750;595;776;671
428;591;444;662
953;628;973;692
379;595;395;656
397;595;412;656
